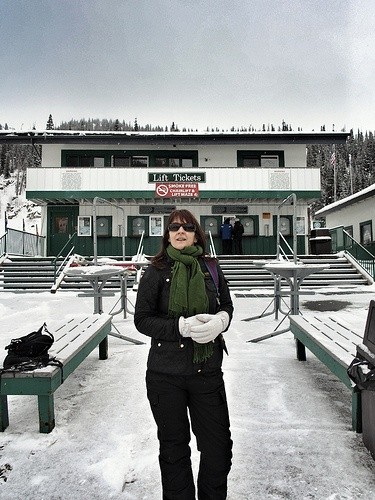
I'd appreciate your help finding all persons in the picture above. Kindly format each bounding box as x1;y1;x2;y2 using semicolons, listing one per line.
133;209;235;500
231;217;245;255
219;218;234;256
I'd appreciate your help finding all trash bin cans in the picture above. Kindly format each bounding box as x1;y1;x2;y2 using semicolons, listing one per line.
346;300;375;460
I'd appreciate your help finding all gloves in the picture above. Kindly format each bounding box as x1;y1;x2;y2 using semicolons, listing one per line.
179;313;215;344
190;310;230;344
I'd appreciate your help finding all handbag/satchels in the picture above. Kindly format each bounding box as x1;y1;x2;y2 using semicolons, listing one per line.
3;322;54;372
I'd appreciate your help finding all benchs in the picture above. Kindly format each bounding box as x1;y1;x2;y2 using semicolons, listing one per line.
290;313;368;432
0;313;112;435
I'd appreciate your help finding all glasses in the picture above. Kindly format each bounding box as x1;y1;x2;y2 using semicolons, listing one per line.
167;222;197;233
